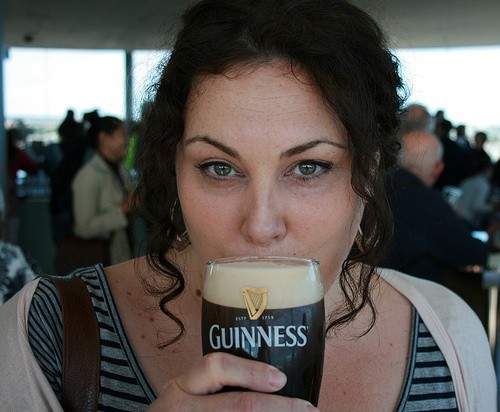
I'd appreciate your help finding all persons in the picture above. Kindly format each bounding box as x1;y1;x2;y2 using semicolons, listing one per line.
0;0;498;412
49;124;88;274
348;131;490;281
71;109;145;266
0;103;499;232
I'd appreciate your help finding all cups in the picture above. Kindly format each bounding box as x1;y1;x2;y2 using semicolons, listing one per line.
201;256;325;407
